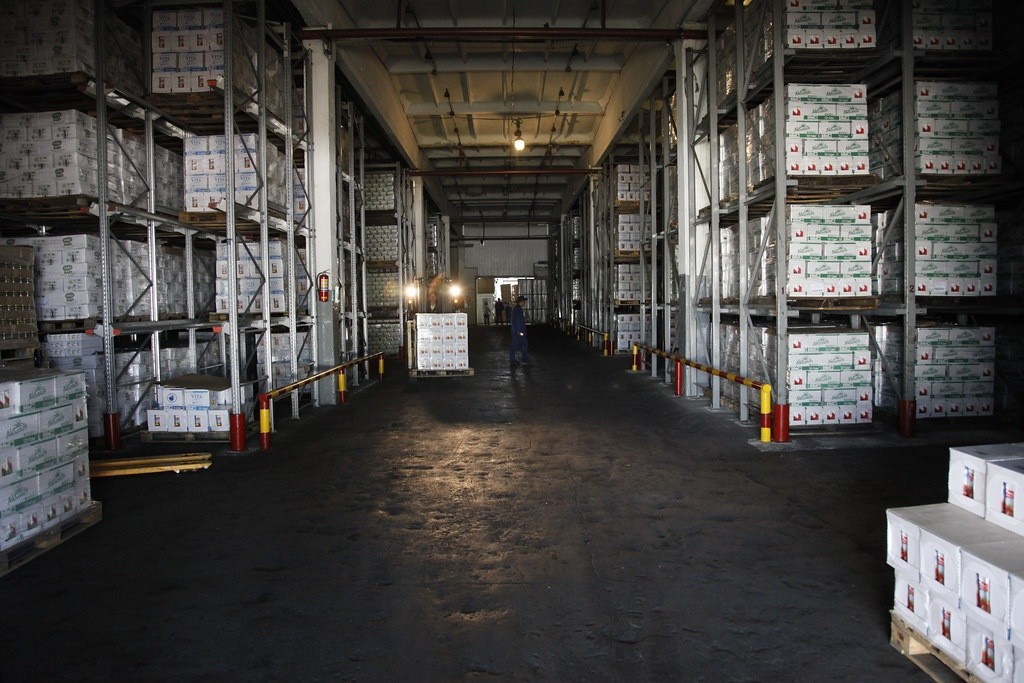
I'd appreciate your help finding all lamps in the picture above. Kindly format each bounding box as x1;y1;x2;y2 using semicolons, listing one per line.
514;117;525;151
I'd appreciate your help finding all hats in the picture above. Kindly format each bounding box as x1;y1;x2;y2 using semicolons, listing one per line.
515;296;528;302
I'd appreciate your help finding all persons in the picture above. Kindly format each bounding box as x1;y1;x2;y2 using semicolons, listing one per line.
505;305;511;325
510;296;528;365
482;302;490;325
495;298;504;325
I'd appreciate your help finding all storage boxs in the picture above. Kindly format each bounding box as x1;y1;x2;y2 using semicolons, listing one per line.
0;0;471;557
555;0;1024;682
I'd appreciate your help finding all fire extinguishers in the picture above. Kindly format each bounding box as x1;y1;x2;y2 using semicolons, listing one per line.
316;269;330;302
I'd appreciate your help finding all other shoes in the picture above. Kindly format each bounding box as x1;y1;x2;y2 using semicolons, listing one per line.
521;359;532;363
509;361;520;365
